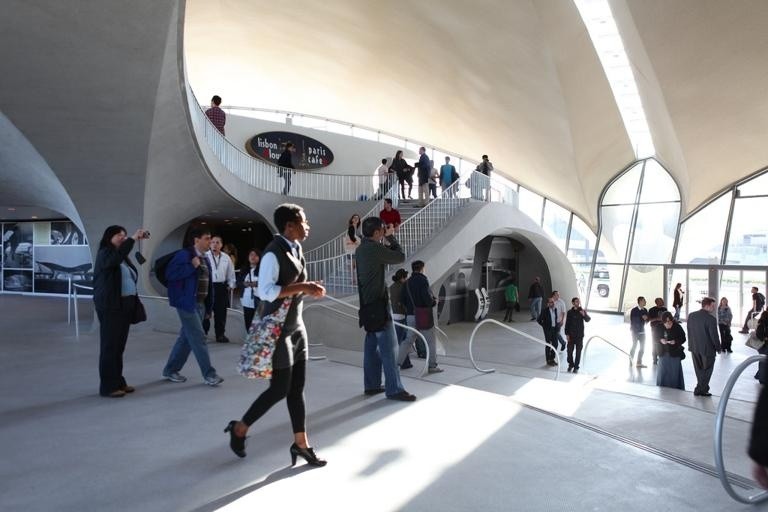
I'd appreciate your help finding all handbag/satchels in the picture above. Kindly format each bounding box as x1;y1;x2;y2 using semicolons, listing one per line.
745;331;766;352
342;235;361;256
117;293;148;325
232;300;292;381
410;298;435;330
357;299;389;334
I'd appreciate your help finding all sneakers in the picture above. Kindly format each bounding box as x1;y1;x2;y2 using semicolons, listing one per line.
568;362;580;373
215;334;230;344
203;374;225;387
721;346;734;354
400;363;413;369
561;344;565;351
628;358;658;368
160;367;188;383
100;384;136;398
547;360;558;366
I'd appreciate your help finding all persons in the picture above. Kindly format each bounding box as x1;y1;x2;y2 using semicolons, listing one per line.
224;204;327;467
343;214;362;284
379;198;402;240
354;217;416;400
628;281;768;396
379;147;494;209
277;142;297;195
155;225;224;387
202;236;238;341
502;278;520;322
237;248;262;334
565;296;592;372
222;240;237;268
397;259;443;372
92;225;152;396
536;297;559;366
527;275;544;320
205;95;226;137
748;364;766;487
388;269;416;369
551;291;566;351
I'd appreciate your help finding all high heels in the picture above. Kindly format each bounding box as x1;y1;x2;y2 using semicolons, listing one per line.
289;442;329;468
222;420;250;458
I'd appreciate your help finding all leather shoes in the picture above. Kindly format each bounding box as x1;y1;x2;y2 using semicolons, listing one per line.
363;383;386;396
387;389;416;401
693;387;714;398
428;363;445;374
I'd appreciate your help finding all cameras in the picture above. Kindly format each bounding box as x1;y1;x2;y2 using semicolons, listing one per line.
143;230;152;241
666;341;670;344
384;222;390;232
486;158;489;162
